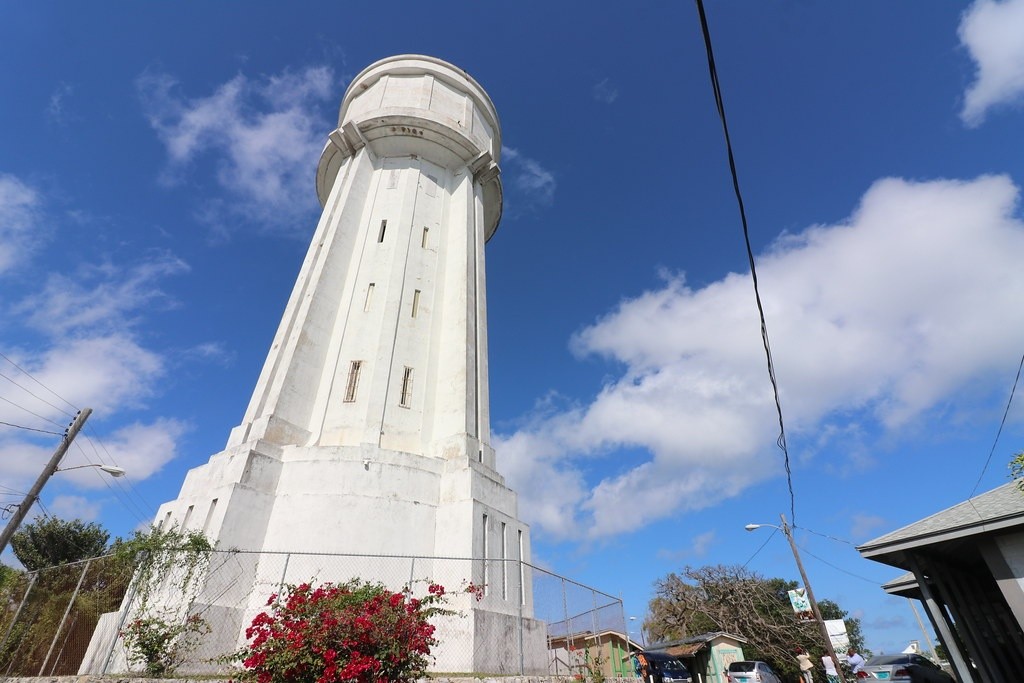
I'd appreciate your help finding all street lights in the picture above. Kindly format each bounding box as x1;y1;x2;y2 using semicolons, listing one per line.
745;524;846;683
0;464;125;552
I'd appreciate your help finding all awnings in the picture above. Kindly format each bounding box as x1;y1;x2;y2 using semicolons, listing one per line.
637;642;705;667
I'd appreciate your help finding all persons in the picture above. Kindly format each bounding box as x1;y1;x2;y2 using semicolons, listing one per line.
847;648;867;683
795;647;814;683
821;649;842;683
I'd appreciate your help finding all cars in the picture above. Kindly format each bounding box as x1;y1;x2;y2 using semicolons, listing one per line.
857;653;955;683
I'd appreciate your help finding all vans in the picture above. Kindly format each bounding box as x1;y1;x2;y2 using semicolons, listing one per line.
634;652;693;683
727;661;781;683
